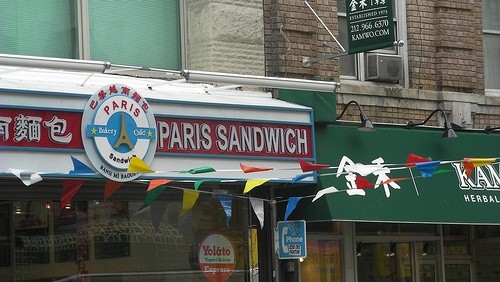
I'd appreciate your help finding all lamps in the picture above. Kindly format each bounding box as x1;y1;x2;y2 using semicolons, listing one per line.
408;107;459;139
337;100;376;133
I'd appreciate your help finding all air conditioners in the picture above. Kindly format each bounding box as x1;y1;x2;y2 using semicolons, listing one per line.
367;52;404;81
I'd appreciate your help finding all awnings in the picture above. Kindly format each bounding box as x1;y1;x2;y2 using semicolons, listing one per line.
303;122;500;226
